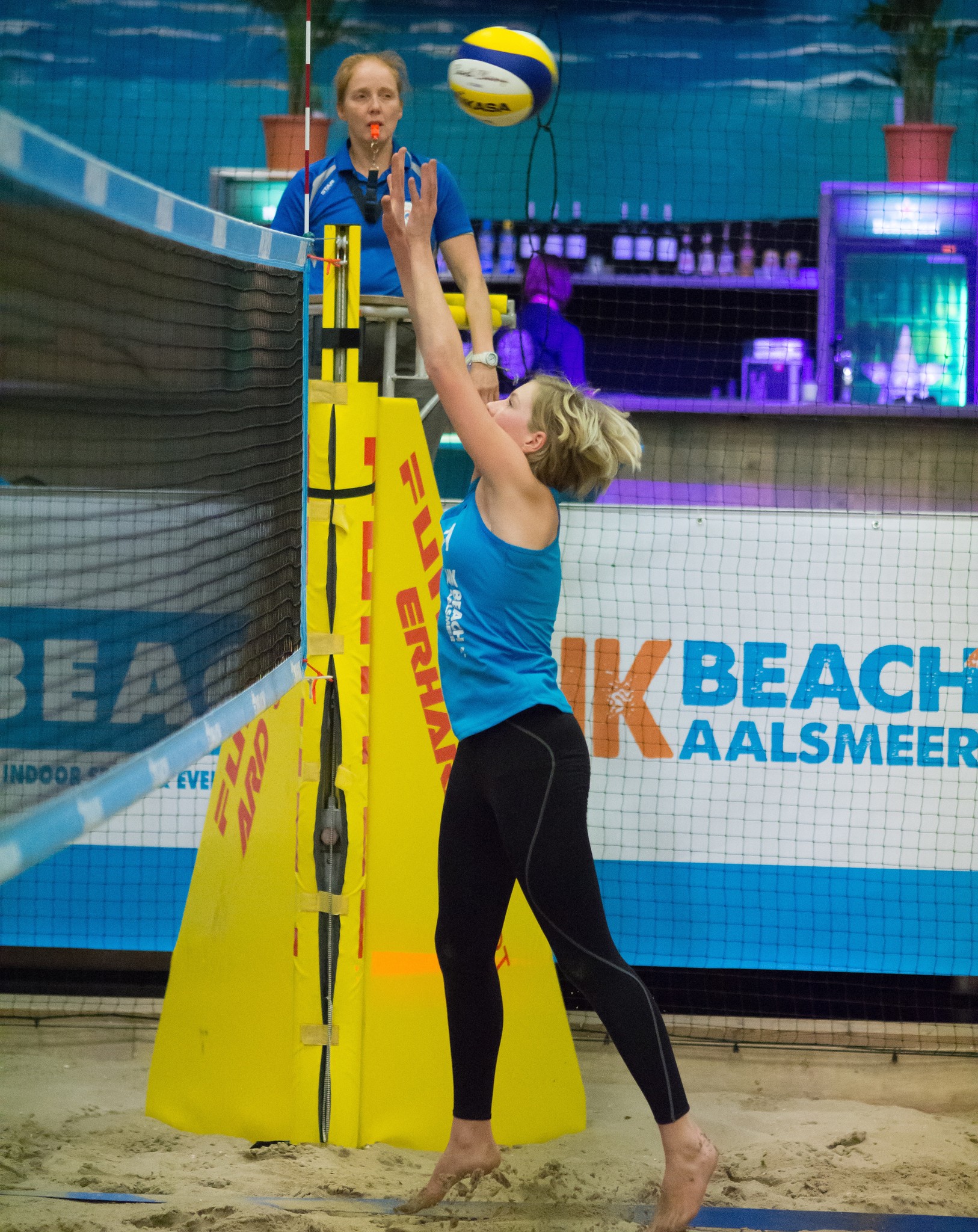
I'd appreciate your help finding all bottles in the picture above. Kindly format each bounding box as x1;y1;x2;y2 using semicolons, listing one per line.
435;200;802;284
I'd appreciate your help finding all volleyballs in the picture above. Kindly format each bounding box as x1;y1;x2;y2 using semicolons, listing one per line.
447;26;559;127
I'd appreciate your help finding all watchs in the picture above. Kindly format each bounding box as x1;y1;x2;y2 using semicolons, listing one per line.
470;351;498;369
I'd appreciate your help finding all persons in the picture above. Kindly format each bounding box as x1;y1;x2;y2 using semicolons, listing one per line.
381;146;719;1232
500;253;586;393
271;53;501;407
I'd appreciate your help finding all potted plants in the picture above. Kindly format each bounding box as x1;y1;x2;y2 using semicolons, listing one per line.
855;0;978;184
249;1;375;170
